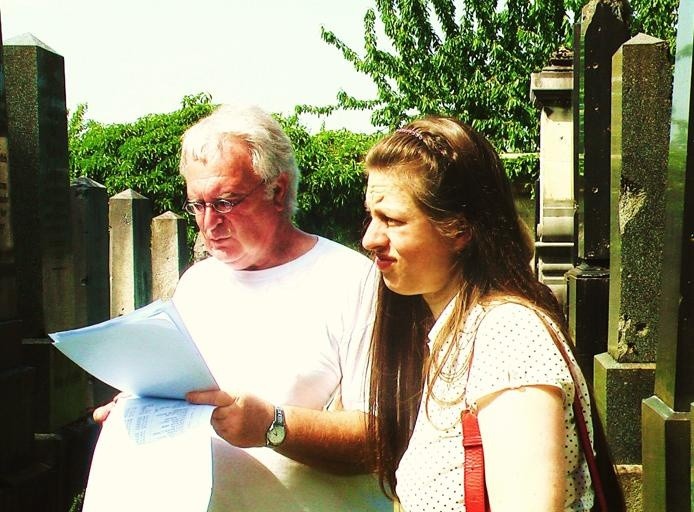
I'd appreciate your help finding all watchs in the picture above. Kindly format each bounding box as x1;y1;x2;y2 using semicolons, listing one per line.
264;404;287;450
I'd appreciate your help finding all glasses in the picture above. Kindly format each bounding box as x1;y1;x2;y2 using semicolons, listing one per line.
181;180;264;215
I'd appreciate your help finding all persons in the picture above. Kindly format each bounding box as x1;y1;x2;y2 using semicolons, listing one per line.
92;104;379;512
362;118;628;511
